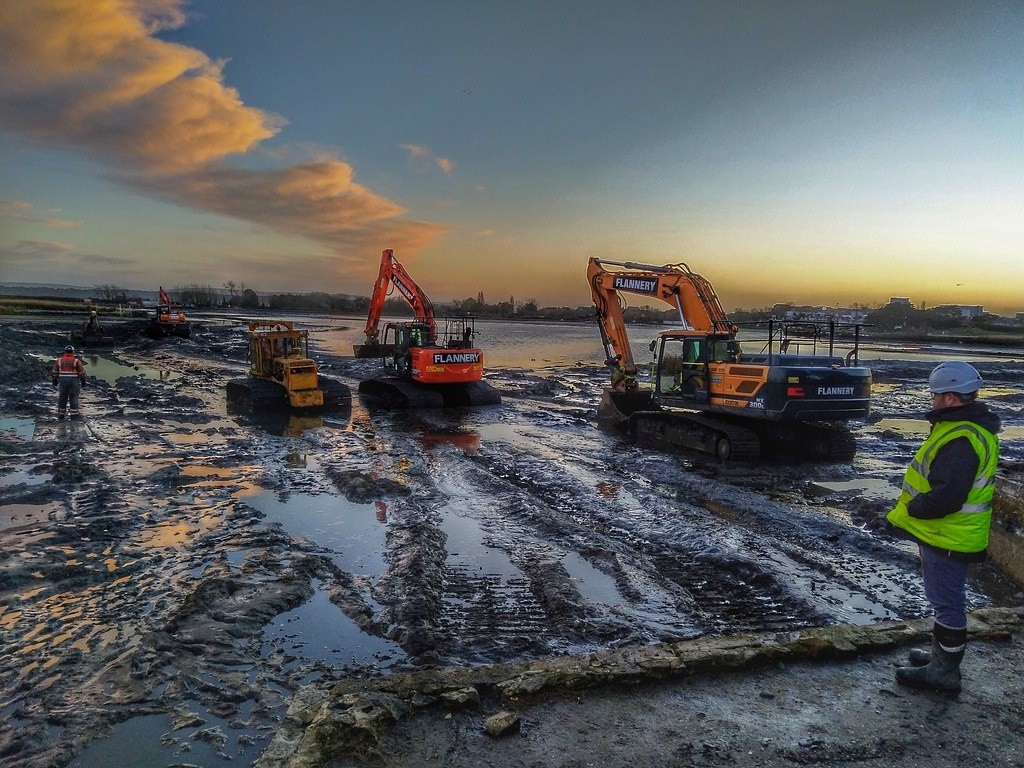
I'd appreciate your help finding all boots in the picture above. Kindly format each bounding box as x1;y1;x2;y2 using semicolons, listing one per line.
58;407;80;421
894;620;967;693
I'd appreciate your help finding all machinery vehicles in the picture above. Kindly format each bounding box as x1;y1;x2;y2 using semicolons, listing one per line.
71;310;114;347
586;255;874;462
353;248;502;408
225;321;352;419
150;285;192;342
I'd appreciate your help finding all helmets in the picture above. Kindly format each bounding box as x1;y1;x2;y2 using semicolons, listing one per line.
64;345;74;351
925;360;989;394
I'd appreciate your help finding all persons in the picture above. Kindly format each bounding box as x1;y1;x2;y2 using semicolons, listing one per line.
53;344;87;422
886;362;1002;688
